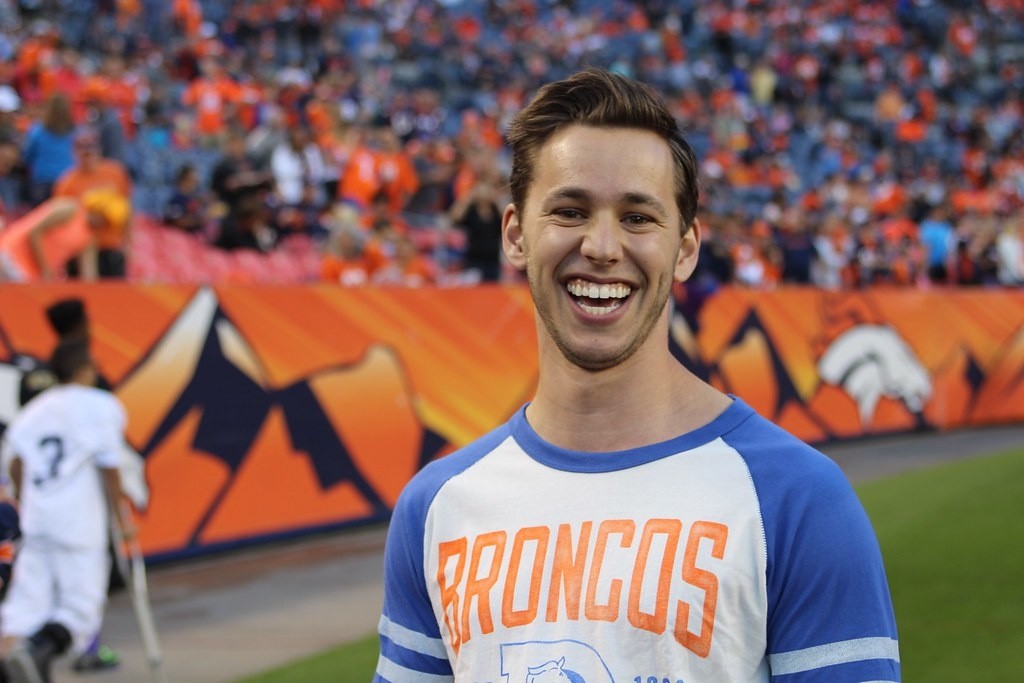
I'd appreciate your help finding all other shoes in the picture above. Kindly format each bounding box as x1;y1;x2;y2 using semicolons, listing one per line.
74;646;119;669
4;630;63;683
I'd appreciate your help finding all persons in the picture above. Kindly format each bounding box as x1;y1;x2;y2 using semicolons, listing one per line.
0;341;148;683
0;0;1024;289
374;70;902;683
